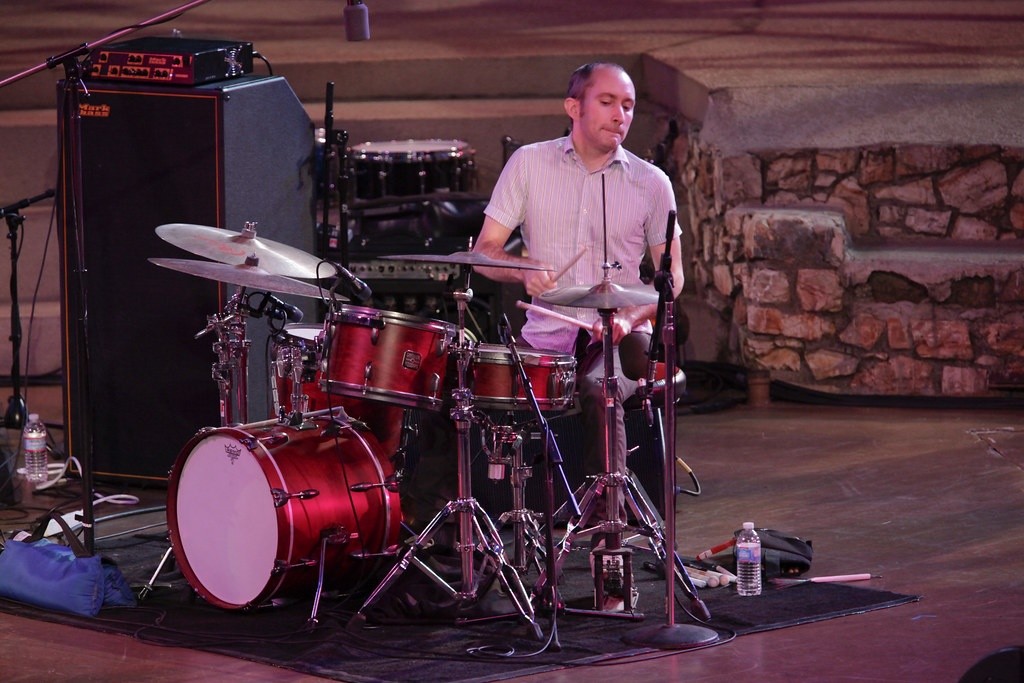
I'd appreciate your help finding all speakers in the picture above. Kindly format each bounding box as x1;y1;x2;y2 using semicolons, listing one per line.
56;75;319;489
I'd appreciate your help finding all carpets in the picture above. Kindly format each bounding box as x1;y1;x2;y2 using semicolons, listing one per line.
0;502;930;683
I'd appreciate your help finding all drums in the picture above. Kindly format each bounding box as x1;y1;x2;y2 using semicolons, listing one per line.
164;305;577;610
339;133;477;188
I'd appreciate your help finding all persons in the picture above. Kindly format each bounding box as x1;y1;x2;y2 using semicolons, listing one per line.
469;59;687;564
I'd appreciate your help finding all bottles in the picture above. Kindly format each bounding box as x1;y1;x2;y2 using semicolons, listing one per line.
737;521;761;596
23;413;48;484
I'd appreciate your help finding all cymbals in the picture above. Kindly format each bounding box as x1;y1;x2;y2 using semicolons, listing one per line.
539;282;659;309
145;221;348;301
379;250;552;273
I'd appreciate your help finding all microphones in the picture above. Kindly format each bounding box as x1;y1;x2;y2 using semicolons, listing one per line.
264;294;303;323
619;334;655;429
335;265;373;301
343;1;369;42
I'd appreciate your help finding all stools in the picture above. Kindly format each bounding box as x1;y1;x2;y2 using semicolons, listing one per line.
523;361;687;540
